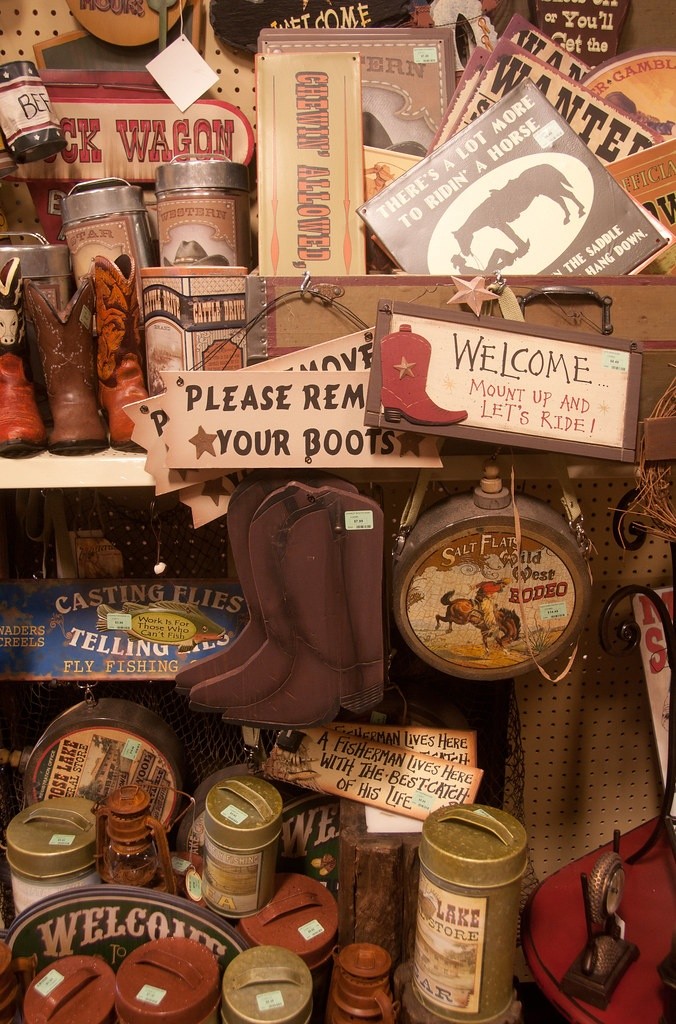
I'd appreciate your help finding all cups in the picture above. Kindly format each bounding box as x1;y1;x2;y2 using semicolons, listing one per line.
0;132;19;178
0;61;68;164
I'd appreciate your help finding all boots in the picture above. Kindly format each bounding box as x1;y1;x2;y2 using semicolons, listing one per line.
0;252;149;456
174;471;384;728
379;325;468;427
